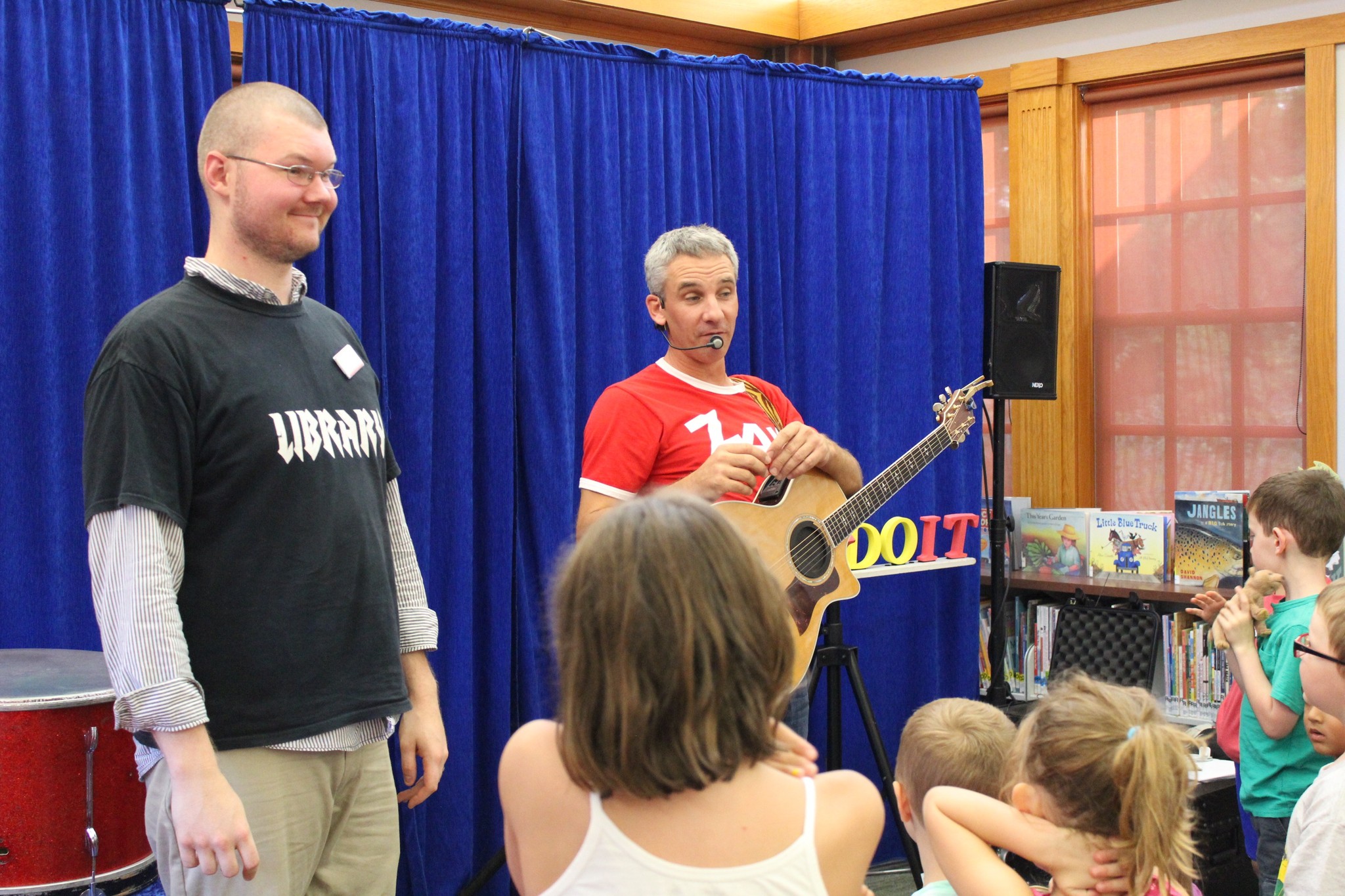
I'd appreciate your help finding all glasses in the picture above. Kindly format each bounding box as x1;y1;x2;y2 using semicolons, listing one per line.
1294;634;1345;665
225;154;345;190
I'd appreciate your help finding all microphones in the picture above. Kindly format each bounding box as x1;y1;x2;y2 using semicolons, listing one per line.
661;328;725;352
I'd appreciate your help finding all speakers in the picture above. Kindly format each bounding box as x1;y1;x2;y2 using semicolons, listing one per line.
983;260;1061;401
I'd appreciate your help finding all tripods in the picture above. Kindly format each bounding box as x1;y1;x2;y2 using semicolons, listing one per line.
801;605;923;892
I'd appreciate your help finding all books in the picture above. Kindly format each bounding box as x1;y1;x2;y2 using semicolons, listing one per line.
1183;754;1236;801
978;596;1235;722
981;491;1251;588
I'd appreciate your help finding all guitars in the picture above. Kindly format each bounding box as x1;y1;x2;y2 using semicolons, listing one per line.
709;380;999;710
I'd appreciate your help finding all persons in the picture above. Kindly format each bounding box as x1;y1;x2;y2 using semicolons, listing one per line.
575;223;864;742
1184;470;1345;896
499;498;886;896
893;670;1208;896
83;80;449;896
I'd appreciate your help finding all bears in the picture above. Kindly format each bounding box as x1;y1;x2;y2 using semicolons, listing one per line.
1206;566;1285;651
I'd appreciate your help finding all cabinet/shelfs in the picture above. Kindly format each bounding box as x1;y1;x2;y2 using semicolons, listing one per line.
979;567;1252;896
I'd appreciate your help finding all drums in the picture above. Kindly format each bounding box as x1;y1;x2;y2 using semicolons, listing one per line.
0;647;166;896
